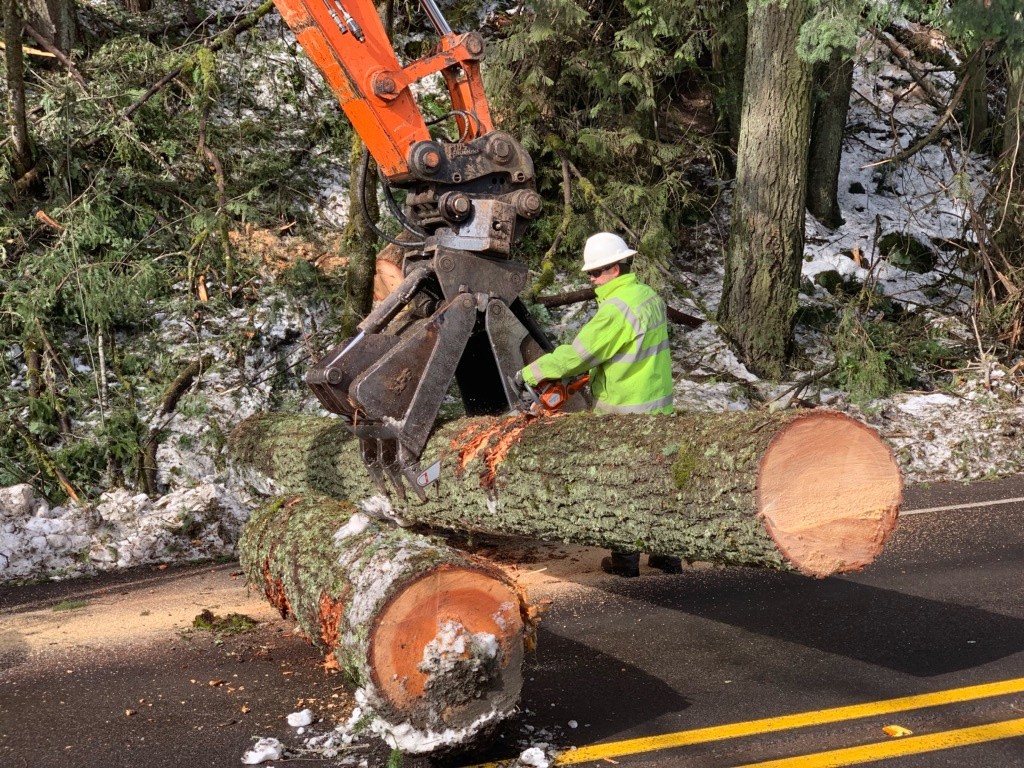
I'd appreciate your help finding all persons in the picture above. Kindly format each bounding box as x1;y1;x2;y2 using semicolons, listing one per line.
515;232;682;577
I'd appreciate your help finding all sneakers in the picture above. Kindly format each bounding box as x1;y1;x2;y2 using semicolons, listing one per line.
600;556;639;577
648;553;681;574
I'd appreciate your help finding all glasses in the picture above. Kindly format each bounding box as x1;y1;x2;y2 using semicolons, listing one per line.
588;262;618;278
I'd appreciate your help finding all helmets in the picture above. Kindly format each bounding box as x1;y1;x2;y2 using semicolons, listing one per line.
581;232;638;272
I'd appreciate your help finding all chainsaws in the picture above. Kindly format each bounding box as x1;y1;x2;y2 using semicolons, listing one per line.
417;380;590;487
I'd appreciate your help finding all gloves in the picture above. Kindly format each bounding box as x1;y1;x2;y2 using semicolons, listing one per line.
514;370;528;392
